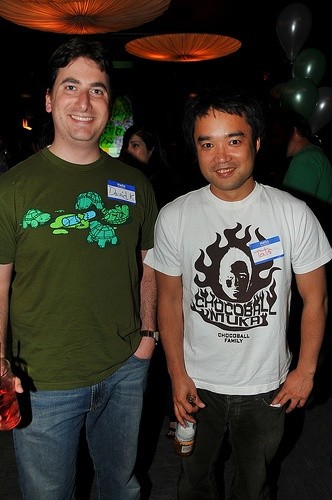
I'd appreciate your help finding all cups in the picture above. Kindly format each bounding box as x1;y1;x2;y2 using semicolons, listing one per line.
0;359;22;431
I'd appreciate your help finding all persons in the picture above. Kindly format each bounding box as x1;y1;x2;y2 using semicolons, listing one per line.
143;83;332;500
0;37;158;500
272;110;332;248
98;78;209;210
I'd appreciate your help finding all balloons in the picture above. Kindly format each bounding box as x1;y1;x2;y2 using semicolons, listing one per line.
281;78;319;120
269;82;288;106
291;48;326;84
276;4;312;62
309;87;332;134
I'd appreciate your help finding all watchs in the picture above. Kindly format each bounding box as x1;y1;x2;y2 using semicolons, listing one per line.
140;330;159;341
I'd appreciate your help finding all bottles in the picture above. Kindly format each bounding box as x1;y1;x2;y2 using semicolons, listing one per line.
172;393;197;457
